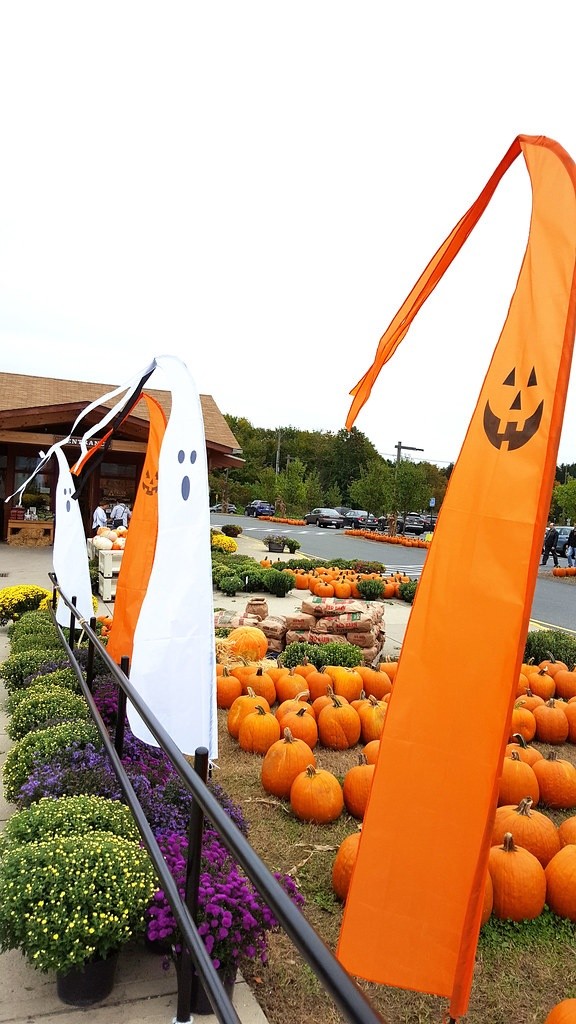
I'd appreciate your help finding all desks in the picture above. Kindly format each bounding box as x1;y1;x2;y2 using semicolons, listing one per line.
7;520;54;545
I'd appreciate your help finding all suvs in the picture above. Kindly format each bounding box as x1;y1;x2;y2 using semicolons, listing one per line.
378;511;425;535
210;503;236;514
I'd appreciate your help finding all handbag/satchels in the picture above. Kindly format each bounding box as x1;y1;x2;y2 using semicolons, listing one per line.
114;519;123;529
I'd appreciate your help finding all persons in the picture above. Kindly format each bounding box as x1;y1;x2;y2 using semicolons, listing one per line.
224;501;229;513
567;517;571;525
277;500;287;519
92;497;132;537
540;521;576;569
388;516;397;537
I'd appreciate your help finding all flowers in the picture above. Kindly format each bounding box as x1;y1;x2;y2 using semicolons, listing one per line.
0;535;307;987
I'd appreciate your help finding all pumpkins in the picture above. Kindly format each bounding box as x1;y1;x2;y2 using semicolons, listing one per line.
480;651;576;928
92;526;129;549
543;998;576;1024
215;626;398;901
98;615;113;636
261;556;412;599
553;565;576;576
345;529;430;548
258;516;307;526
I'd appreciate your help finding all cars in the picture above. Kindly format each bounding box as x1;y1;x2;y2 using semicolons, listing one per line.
335;507;352;514
244;499;275;517
420;514;438;531
542;526;575;558
304;508;345;529
344;510;378;530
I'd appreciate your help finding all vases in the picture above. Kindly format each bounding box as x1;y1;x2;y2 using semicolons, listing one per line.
263;586;269;592
146;933;178;953
226;533;237;537
276;590;285;597
57;955;116;1005
268;542;284;552
227;591;236;596
174;964;236;1015
290;548;295;554
